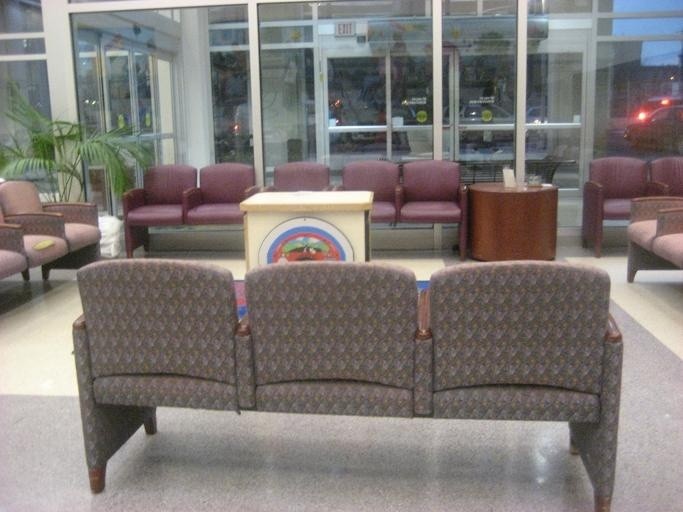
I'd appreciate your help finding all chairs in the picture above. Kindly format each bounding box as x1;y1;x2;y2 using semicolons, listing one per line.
265;162;334;192
120;163;259;258
69;255;624;512
580;154;681;286
0;177;102;300
340;159;465;254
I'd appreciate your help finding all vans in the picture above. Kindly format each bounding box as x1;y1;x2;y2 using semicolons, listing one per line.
640;95;682;119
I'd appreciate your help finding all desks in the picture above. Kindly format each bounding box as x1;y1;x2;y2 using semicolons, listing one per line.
465;180;558;262
237;188;375;271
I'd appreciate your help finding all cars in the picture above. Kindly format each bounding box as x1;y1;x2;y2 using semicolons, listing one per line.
622;102;682;154
